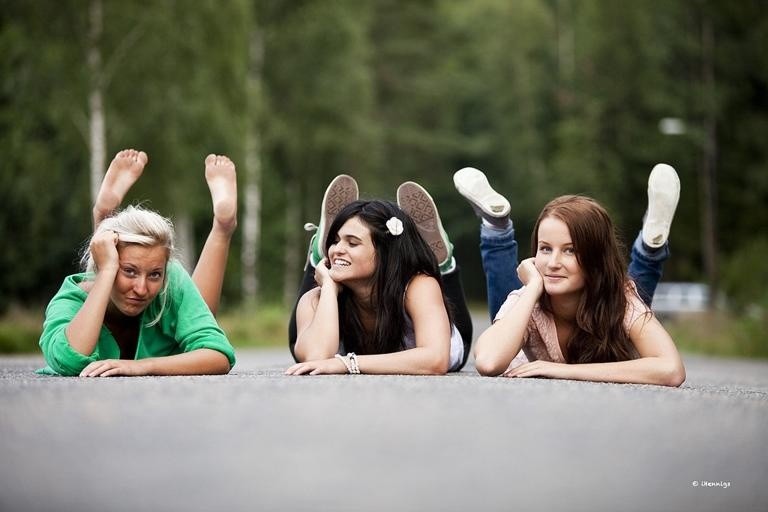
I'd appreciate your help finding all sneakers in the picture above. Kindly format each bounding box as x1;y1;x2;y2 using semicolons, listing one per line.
309;174;360;266
451;166;511;229
641;162;681;251
395;180;453;273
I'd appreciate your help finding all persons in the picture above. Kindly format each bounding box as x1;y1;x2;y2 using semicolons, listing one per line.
285;174;473;377
34;150;241;379
453;163;687;387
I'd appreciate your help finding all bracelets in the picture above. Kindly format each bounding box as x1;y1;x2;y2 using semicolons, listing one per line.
334;352;363;374
